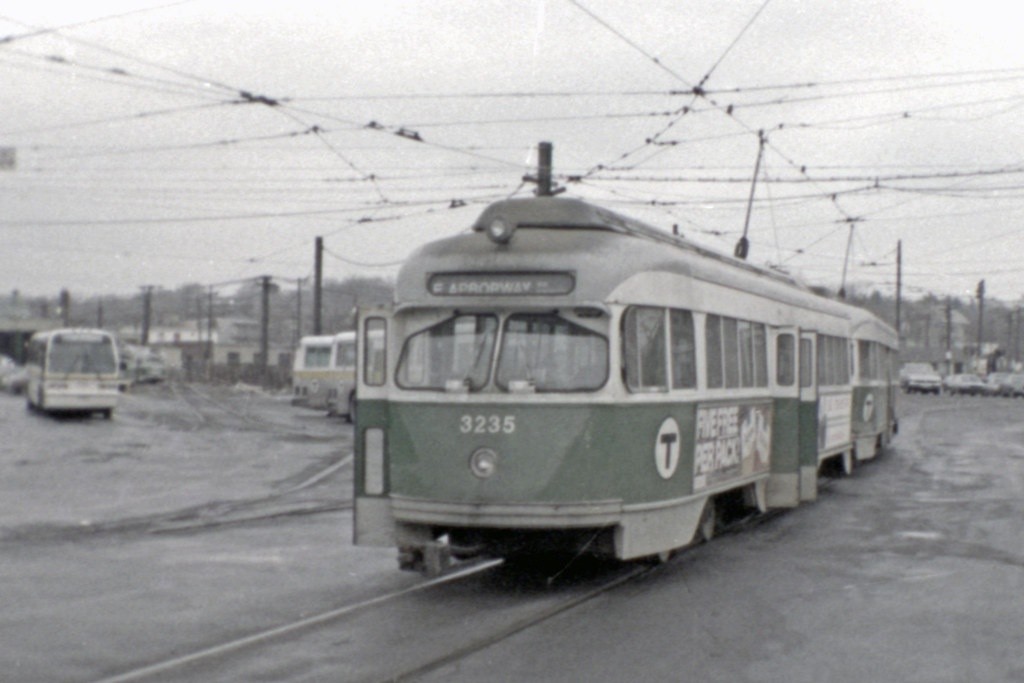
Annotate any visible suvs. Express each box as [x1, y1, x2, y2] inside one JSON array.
[[900, 361, 941, 395]]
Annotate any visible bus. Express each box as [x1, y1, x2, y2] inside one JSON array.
[[290, 325, 500, 426], [23, 327, 120, 419], [341, 192, 904, 575]]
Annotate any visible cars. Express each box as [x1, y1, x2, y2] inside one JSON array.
[[940, 373, 1024, 397]]
